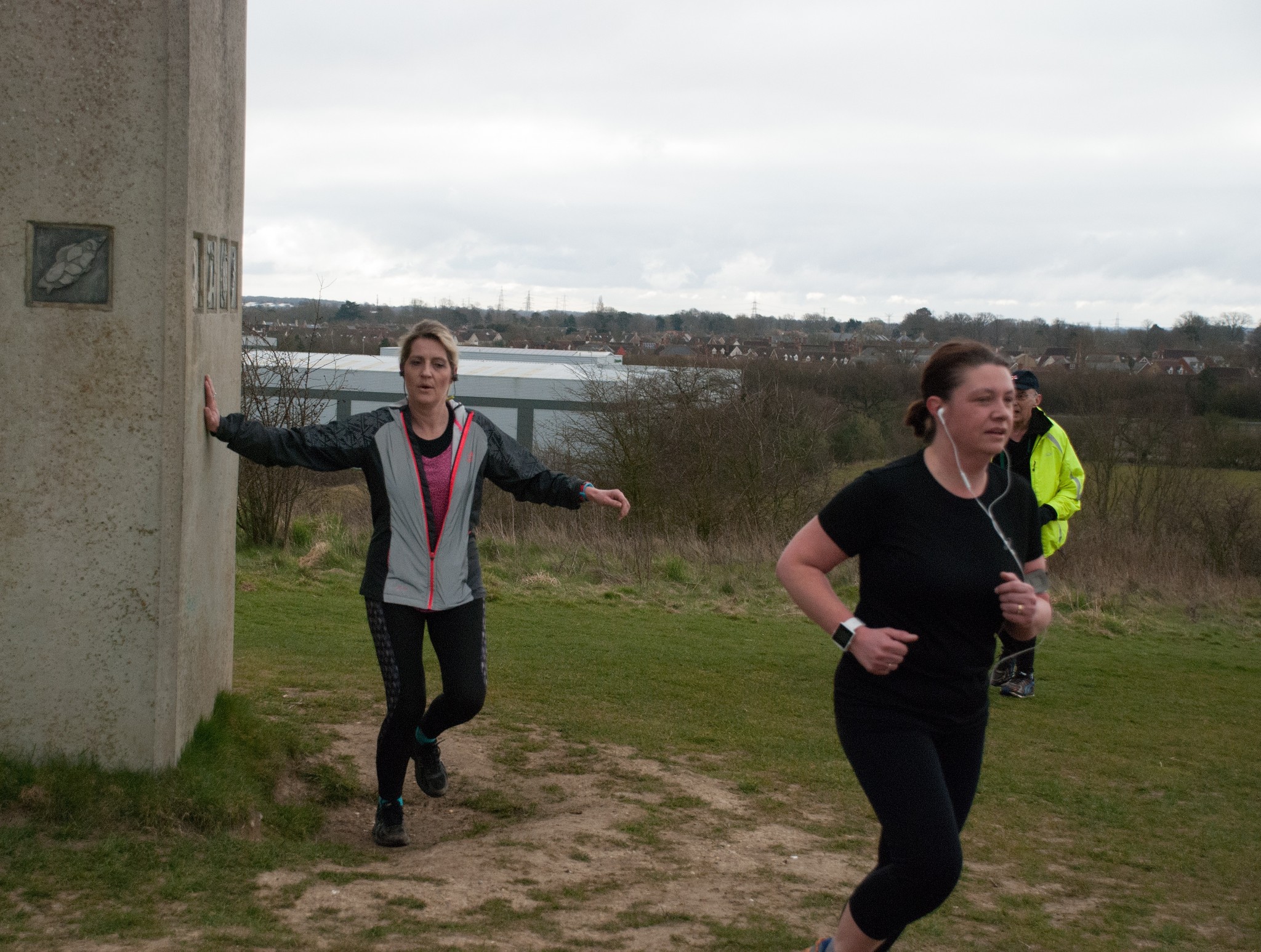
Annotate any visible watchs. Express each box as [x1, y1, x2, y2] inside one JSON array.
[[830, 616, 867, 652]]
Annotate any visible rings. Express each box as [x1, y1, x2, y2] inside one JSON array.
[[1017, 604, 1023, 615], [886, 663, 892, 671]]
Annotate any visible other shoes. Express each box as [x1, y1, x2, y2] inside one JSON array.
[[804, 936, 832, 952]]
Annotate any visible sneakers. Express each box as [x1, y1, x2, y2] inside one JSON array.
[[1000, 670, 1035, 698], [989, 646, 1016, 686], [372, 795, 409, 847], [410, 727, 448, 798]]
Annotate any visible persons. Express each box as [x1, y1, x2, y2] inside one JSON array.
[[990, 370, 1086, 699], [776, 341, 1055, 950], [203, 319, 632, 846]]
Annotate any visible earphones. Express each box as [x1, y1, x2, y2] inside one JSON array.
[[937, 407, 944, 426], [399, 370, 405, 376], [451, 374, 457, 382]]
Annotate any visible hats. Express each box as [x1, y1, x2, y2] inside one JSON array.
[[1011, 370, 1039, 390]]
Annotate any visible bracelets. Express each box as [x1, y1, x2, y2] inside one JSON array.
[[579, 481, 593, 502]]
